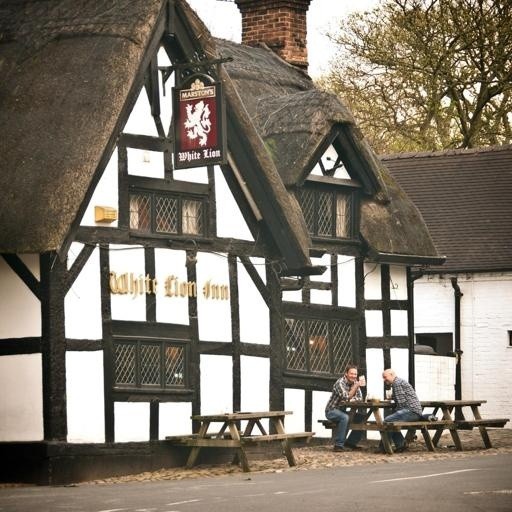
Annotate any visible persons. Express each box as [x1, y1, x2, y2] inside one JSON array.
[[325, 364, 368, 452], [372, 368, 424, 454]]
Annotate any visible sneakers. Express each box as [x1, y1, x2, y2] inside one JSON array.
[[332, 440, 361, 452], [375, 440, 408, 453]]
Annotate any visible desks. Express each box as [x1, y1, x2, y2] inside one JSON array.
[[186, 411, 296, 473], [339, 399, 492, 456]]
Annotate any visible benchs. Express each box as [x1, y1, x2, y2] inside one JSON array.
[[317, 417, 438, 443], [382, 418, 510, 455], [240, 431, 316, 472], [165, 431, 243, 470]]
